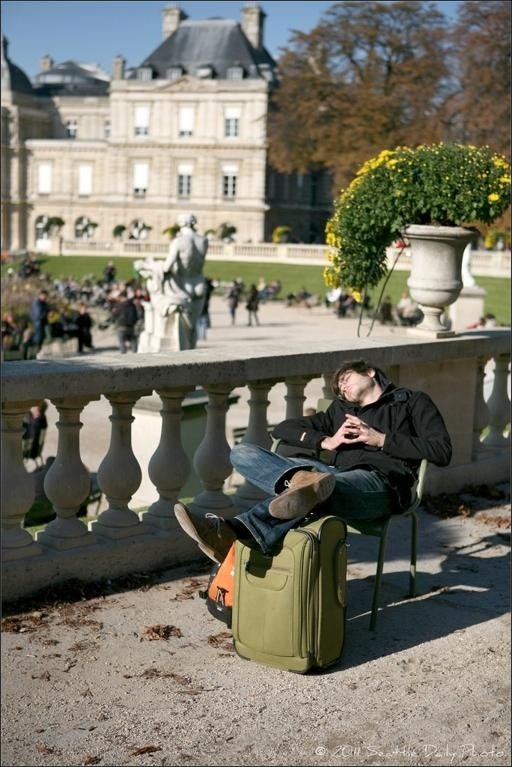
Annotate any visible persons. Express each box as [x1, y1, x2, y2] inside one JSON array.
[[301, 293, 320, 308], [245, 283, 261, 325], [287, 285, 311, 306], [165, 210, 209, 301], [322, 285, 424, 327], [195, 277, 215, 340], [1, 251, 150, 359], [256, 274, 267, 306], [235, 275, 243, 292], [225, 280, 238, 325], [172, 359, 452, 568], [262, 278, 281, 304]]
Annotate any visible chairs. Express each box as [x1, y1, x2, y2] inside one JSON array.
[[345, 459, 428, 631]]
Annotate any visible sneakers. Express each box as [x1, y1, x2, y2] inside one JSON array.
[[268, 470, 335, 520], [173, 502, 236, 565]]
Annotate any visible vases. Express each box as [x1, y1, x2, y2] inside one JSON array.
[[401, 222, 475, 339]]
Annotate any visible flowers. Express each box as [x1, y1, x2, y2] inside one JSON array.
[[324, 142, 512, 303]]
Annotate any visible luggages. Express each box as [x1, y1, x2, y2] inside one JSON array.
[[207, 512, 345, 678]]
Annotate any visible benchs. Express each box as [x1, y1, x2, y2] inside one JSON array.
[[233, 425, 278, 445], [21, 471, 103, 540]]
